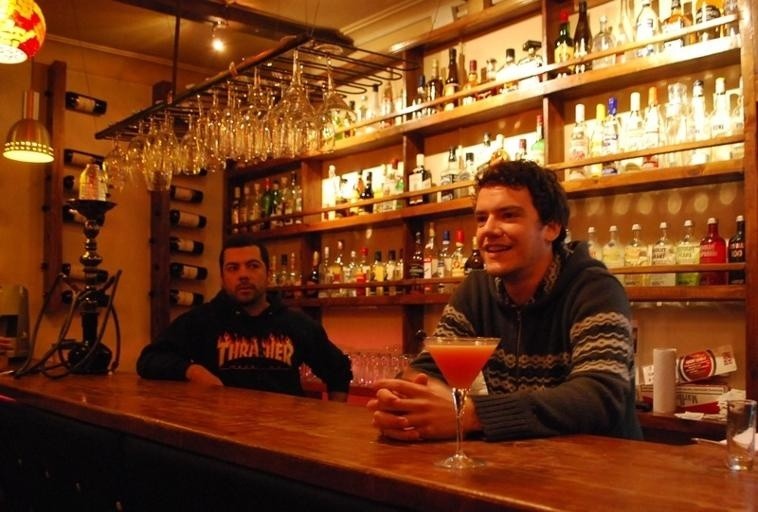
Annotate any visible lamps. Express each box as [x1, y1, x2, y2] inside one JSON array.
[[3, 91, 56, 164]]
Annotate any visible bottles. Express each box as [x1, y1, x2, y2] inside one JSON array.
[[42, 91, 112, 307], [357, 75, 401, 132], [357, 227, 485, 294], [409, 38, 544, 116], [410, 112, 545, 204], [302, 347, 409, 385], [586, 198, 745, 290], [552, 5, 743, 76], [230, 167, 374, 299], [565, 74, 743, 176], [375, 157, 403, 212], [150, 186, 208, 308]]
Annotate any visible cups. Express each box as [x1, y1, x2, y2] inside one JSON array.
[[725, 400, 757, 471]]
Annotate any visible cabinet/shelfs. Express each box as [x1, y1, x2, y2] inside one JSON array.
[[222, 0, 758, 448]]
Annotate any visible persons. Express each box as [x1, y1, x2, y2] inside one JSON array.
[[137, 235, 353, 403], [367, 162, 644, 444]]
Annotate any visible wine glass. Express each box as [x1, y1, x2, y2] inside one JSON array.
[[102, 51, 356, 191], [424, 335, 504, 471]]
